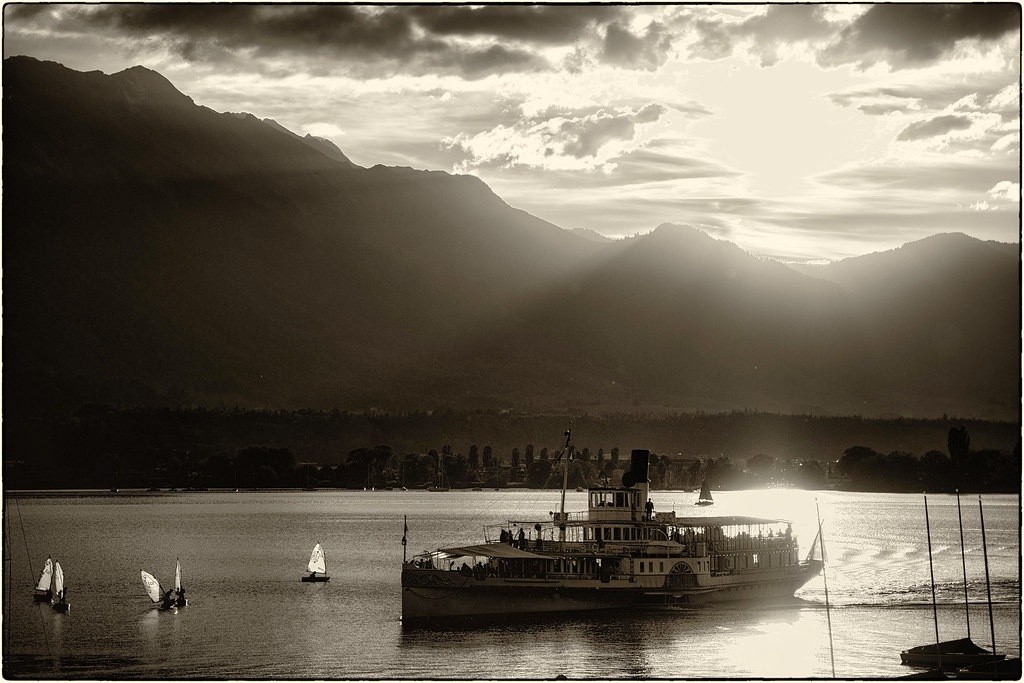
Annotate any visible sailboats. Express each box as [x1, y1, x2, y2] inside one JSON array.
[[53, 560, 71, 613], [695, 476, 714, 506], [428, 462, 450, 492], [34, 555, 53, 603], [139, 569, 177, 612], [472, 476, 482, 491], [494, 469, 499, 491], [301, 543, 330, 583], [402, 468, 407, 491], [173, 557, 187, 607]]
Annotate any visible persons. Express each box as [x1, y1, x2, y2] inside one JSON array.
[[675, 529, 681, 544], [757, 531, 763, 538], [768, 528, 774, 537], [785, 525, 792, 536], [500, 529, 514, 547], [669, 528, 675, 540], [519, 528, 525, 551], [645, 498, 654, 523], [776, 529, 784, 537], [414, 557, 546, 582]]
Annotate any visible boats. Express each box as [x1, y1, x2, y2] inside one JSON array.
[[900, 636, 1005, 667], [577, 487, 584, 492], [401, 428, 825, 629]]
